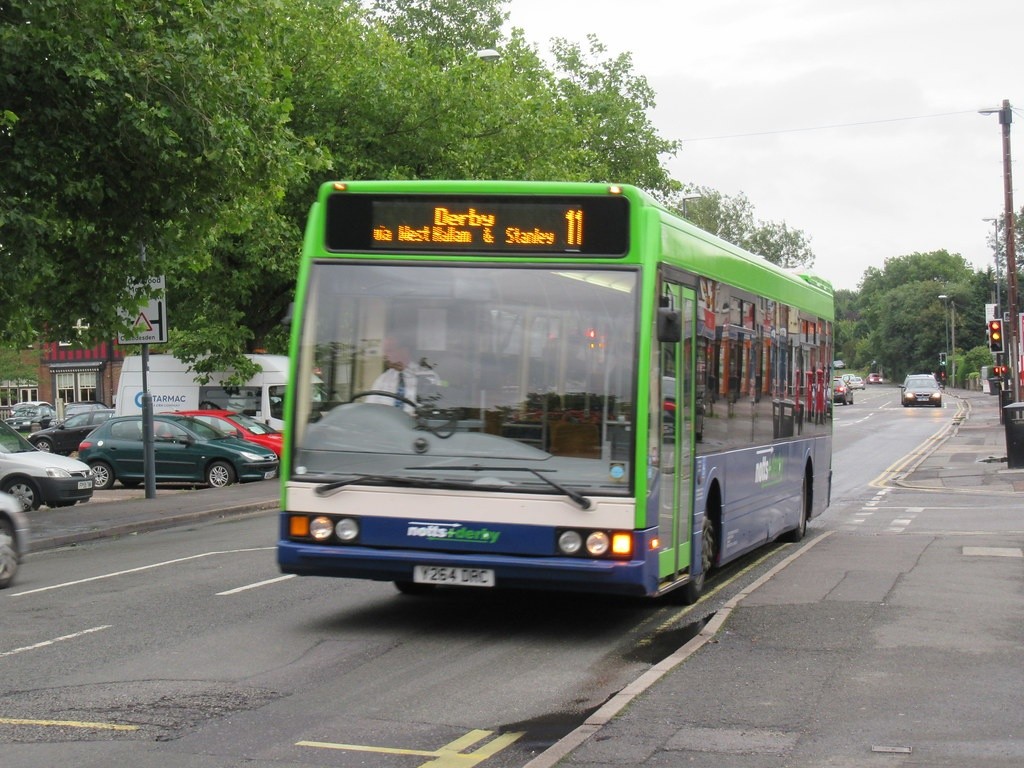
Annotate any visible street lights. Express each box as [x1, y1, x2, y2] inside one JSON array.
[[938, 294, 949, 386], [982, 218, 1005, 365]]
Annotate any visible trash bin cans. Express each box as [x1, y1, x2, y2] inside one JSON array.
[[1003, 401, 1024, 469], [999, 390, 1012, 425], [950, 375, 958, 388], [988, 378, 1000, 394]]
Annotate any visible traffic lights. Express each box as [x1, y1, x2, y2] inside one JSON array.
[[585, 327, 597, 350], [987, 319, 1005, 353], [993, 364, 1008, 377], [939, 353, 947, 366]]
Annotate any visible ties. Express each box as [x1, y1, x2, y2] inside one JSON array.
[[395, 372, 405, 408]]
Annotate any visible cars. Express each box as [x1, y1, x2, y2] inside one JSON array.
[[64, 402, 110, 419], [77, 414, 279, 487], [898, 374, 942, 408], [834, 360, 845, 370], [847, 377, 866, 391], [662, 375, 705, 444], [154, 409, 284, 461], [4, 406, 57, 431], [833, 376, 854, 405], [866, 373, 883, 384], [10, 401, 53, 415], [27, 408, 117, 457], [841, 374, 855, 383], [0, 490, 32, 590]]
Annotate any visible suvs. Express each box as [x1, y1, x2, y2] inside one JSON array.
[[0, 416, 94, 513]]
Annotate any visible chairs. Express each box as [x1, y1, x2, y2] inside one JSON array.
[[549, 410, 600, 459]]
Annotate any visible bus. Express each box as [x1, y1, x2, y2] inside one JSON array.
[[272, 179, 834, 606]]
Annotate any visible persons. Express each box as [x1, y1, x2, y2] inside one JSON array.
[[364, 328, 449, 417]]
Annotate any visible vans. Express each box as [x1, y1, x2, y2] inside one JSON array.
[[114, 352, 347, 436]]
